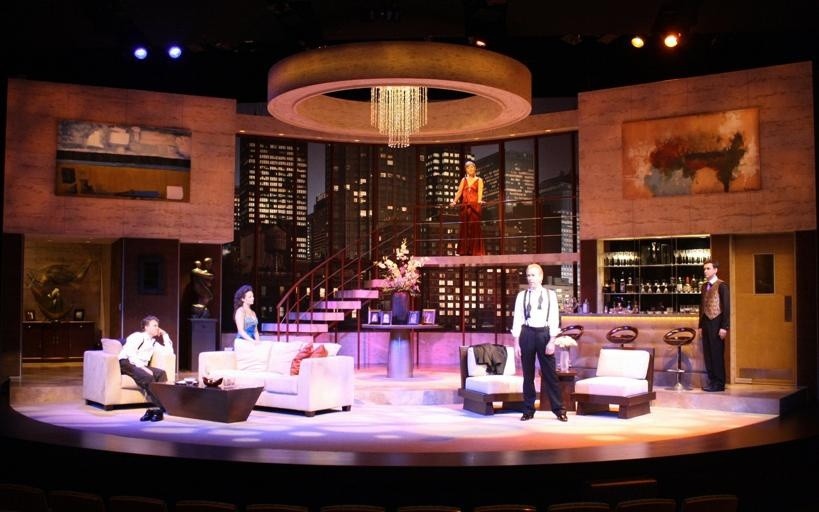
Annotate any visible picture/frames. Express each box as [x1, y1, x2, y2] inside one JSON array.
[[368, 309, 381, 325], [381, 311, 392, 325], [407, 310, 420, 324], [422, 309, 436, 325]]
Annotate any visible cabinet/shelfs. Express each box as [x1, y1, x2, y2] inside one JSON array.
[[580, 234, 712, 315]]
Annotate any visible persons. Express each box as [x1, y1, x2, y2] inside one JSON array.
[[449, 158, 485, 256], [233, 285, 260, 341], [119, 315, 174, 415], [510, 263, 569, 422], [697, 260, 730, 392]]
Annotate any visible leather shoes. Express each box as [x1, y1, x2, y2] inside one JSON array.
[[521, 414, 533, 420], [703, 386, 724, 391], [559, 414, 567, 421], [141, 410, 163, 421]]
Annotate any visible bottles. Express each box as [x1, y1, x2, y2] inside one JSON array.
[[577, 297, 589, 314], [604, 267, 639, 293], [677, 274, 708, 293], [604, 296, 638, 314]]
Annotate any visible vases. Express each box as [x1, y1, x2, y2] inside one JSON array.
[[560, 350, 570, 373], [391, 290, 410, 324]]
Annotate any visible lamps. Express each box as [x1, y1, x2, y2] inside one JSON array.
[[371, 86, 428, 149]]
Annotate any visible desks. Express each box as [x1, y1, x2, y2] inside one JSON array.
[[187, 318, 219, 372], [20, 321, 96, 361], [362, 324, 444, 379]]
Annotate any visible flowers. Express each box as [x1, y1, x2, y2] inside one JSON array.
[[641, 127, 747, 196], [553, 336, 578, 352], [373, 236, 424, 297]]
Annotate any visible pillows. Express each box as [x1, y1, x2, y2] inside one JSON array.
[[233, 338, 341, 376], [101, 337, 123, 354]]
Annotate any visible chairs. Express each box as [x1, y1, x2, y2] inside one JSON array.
[[458, 345, 541, 416], [84, 351, 176, 411], [663, 327, 696, 391], [570, 346, 656, 419], [607, 326, 638, 349]]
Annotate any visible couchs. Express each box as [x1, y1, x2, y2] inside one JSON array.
[[198, 350, 354, 417]]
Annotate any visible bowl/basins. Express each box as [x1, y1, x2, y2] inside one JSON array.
[[184, 378, 197, 385]]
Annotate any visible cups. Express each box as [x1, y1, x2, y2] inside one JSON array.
[[223, 373, 236, 386], [642, 306, 673, 314], [678, 305, 700, 314]]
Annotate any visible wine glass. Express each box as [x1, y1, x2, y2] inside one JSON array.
[[563, 298, 575, 314], [605, 251, 637, 265], [673, 248, 711, 265], [640, 280, 670, 294]]
